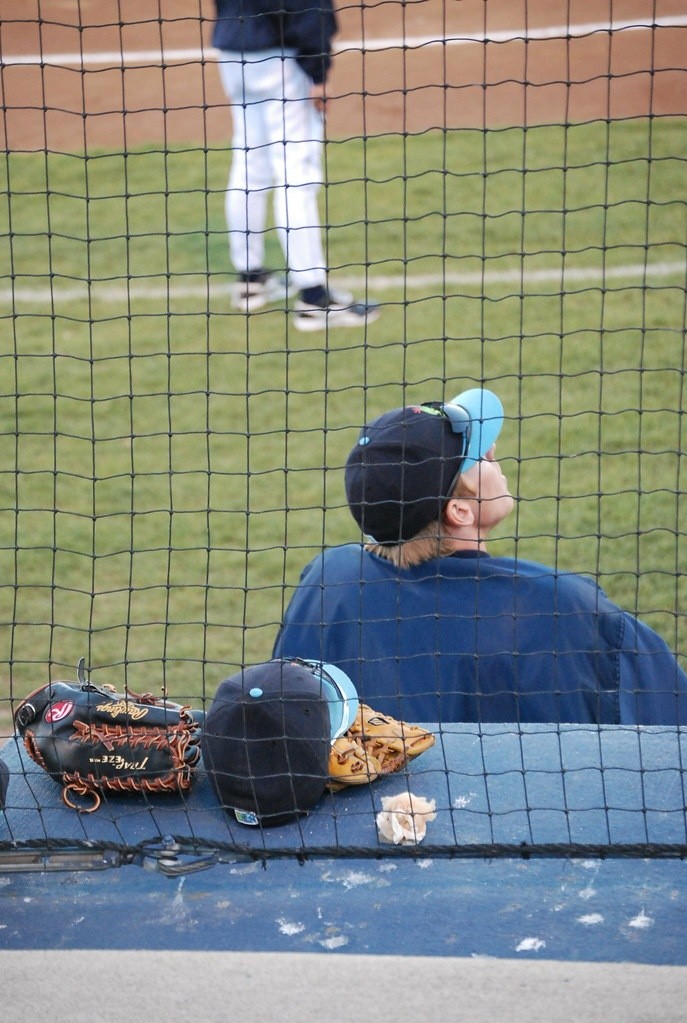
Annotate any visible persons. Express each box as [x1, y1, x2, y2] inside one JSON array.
[[211, 0, 383, 332], [272, 388, 686, 724]]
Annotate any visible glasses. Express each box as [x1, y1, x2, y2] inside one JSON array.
[[420, 401, 470, 522]]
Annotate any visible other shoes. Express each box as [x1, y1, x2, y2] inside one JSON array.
[[292, 289, 381, 332], [231, 272, 301, 312]]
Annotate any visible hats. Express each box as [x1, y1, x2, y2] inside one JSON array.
[[344, 389, 504, 546], [201, 657, 359, 830]]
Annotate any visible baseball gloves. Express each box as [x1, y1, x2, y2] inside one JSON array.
[[327, 704, 435, 796], [13, 656, 208, 813]]
